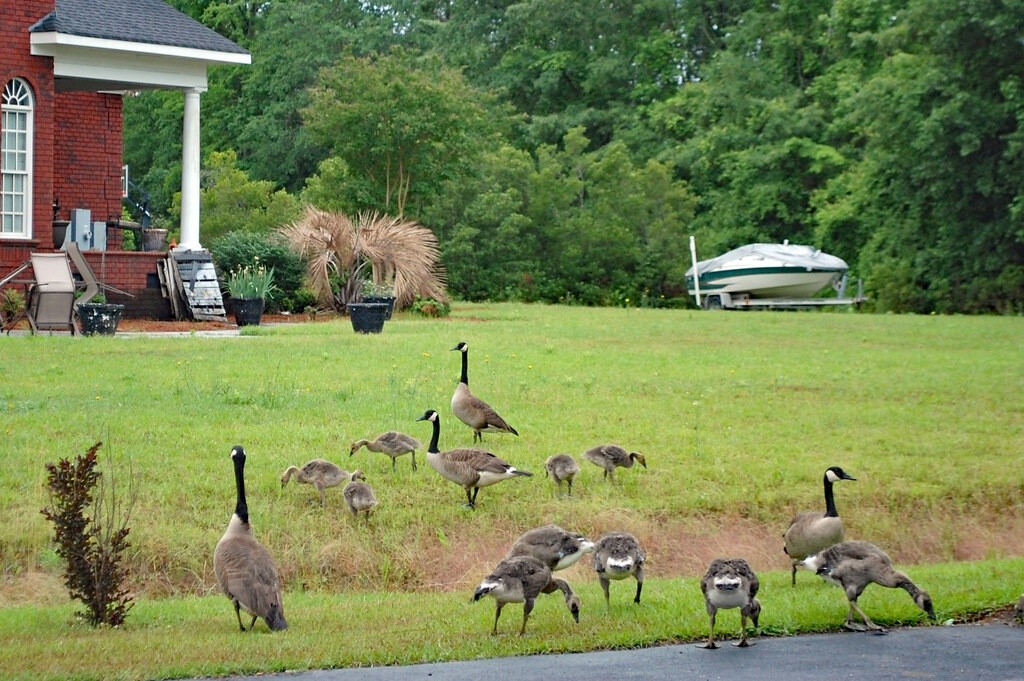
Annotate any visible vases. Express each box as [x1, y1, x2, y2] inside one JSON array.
[[76, 302, 123, 336], [348, 303, 388, 333], [144, 228, 167, 252], [228, 297, 262, 326], [363, 296, 395, 321], [53, 220, 72, 248]]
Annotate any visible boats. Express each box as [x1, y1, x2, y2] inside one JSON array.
[[684, 236, 868, 313]]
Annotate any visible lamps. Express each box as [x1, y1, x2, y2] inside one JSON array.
[[124, 88, 141, 97]]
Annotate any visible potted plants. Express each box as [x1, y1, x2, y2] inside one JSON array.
[[0, 288, 24, 323]]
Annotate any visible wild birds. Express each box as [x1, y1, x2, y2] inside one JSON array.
[[281, 459, 350, 504], [450, 342, 519, 445], [415, 410, 534, 509], [471, 525, 645, 638], [213, 445, 288, 632], [584, 444, 646, 484], [694, 558, 761, 647], [802, 541, 937, 632], [343, 470, 377, 529], [545, 452, 580, 496], [350, 432, 423, 471], [784, 467, 858, 590]]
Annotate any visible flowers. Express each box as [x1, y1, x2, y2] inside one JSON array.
[[360, 278, 394, 297], [218, 256, 284, 302]]
